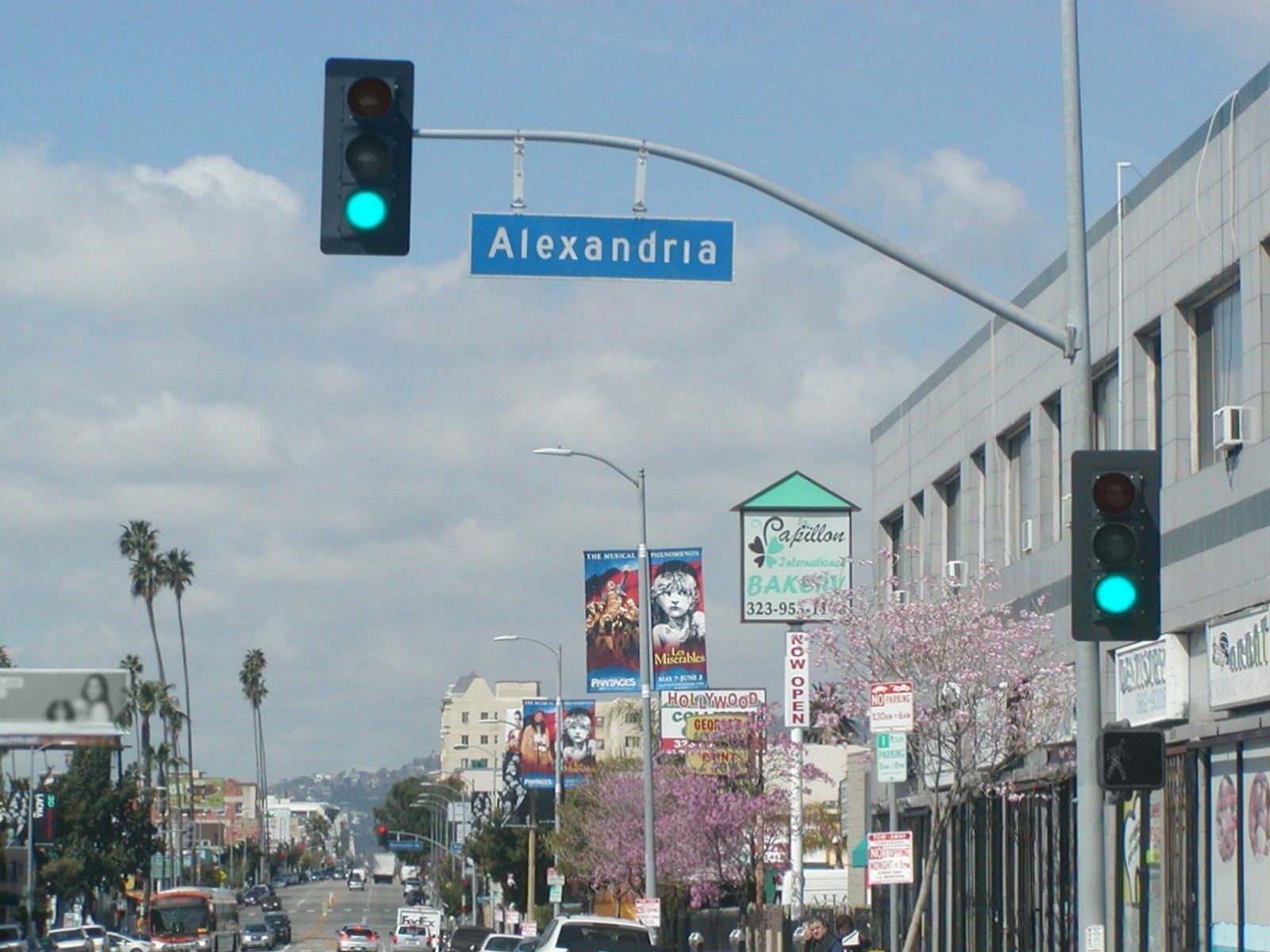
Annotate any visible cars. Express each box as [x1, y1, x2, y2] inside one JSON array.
[[0, 923, 150, 952], [240, 885, 291, 952], [273, 867, 368, 892], [439, 914, 659, 952], [389, 866, 445, 951]]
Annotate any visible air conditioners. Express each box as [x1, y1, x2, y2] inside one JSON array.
[[946, 561, 961, 588], [1213, 406, 1243, 450], [893, 590, 906, 606]]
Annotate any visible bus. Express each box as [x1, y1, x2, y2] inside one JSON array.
[[146, 886, 241, 952]]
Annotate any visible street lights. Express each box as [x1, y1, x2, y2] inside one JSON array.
[[491, 634, 562, 920], [26, 741, 79, 924], [409, 720, 502, 926], [534, 446, 660, 948]]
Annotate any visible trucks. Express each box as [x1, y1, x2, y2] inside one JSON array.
[[370, 854, 398, 883]]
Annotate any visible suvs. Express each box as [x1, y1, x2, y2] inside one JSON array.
[[335, 923, 381, 950]]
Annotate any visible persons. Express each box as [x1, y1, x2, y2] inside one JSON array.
[[734, 926, 745, 952], [805, 916, 843, 952], [773, 873, 782, 905]]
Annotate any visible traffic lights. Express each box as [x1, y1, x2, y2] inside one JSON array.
[[1098, 729, 1169, 789], [376, 825, 388, 849], [280, 851, 285, 861], [320, 57, 416, 255], [1069, 449, 1161, 641]]
